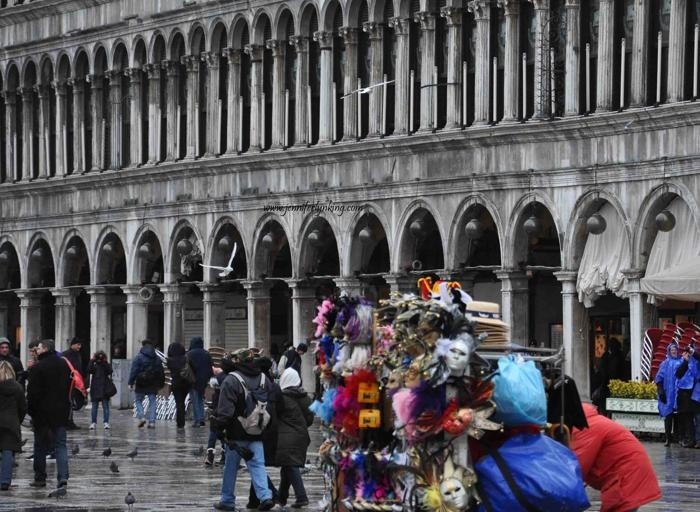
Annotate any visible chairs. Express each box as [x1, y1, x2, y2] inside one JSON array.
[[641, 323, 699, 394], [133, 339, 227, 420]]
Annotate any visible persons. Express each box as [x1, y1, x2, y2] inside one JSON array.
[[0, 329, 328, 512], [654, 342, 700, 449], [567, 400, 664, 512]]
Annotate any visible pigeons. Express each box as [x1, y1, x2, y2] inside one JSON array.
[[101, 447, 112, 459], [124, 491, 136, 510], [48, 481, 69, 501], [127, 446, 139, 461], [109, 461, 119, 474], [71, 443, 81, 456], [191, 445, 204, 458]]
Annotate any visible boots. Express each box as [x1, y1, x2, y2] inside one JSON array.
[[664, 439, 669, 447], [66, 419, 81, 431], [204, 447, 226, 466]]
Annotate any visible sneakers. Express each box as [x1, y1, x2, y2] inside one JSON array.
[[30, 480, 46, 487], [45, 453, 57, 463], [25, 454, 35, 461], [137, 418, 146, 427], [246, 488, 288, 511], [104, 422, 110, 429], [677, 439, 699, 448], [147, 421, 155, 428], [88, 422, 96, 429], [177, 427, 184, 431], [56, 479, 67, 488], [192, 423, 200, 428], [213, 501, 236, 512], [0, 482, 10, 491], [291, 499, 309, 507], [200, 421, 205, 426]]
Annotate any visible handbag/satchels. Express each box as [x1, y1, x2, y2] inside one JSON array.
[[179, 353, 197, 385], [102, 373, 117, 398], [135, 353, 158, 395]]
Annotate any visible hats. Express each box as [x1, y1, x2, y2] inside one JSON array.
[[0, 337, 10, 344], [298, 343, 308, 352], [71, 337, 83, 345], [142, 339, 152, 346], [237, 349, 254, 362], [99, 350, 103, 355]]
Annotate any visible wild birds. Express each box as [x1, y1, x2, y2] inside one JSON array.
[[338, 79, 396, 101], [196, 241, 239, 282]]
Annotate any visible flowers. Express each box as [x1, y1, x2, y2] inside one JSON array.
[[606, 374, 663, 401]]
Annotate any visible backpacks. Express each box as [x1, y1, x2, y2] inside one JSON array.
[[60, 355, 88, 411], [228, 371, 272, 436]]
